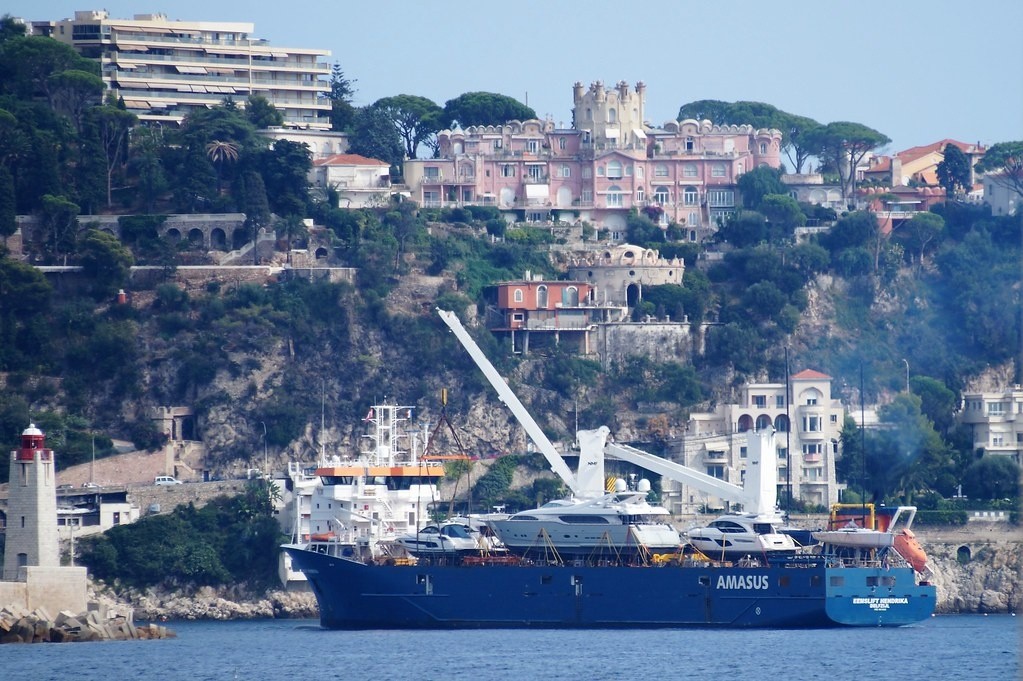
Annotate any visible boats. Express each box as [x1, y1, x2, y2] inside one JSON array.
[[393, 516, 506, 558], [277, 311, 937, 629], [480, 491, 681, 563], [812, 526, 895, 549], [686, 516, 802, 562]]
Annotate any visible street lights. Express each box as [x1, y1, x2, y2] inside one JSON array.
[[902, 358, 909, 393]]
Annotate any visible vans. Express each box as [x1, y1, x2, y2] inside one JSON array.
[[154, 477, 182, 486], [81, 483, 101, 488], [56, 485, 73, 490]]
